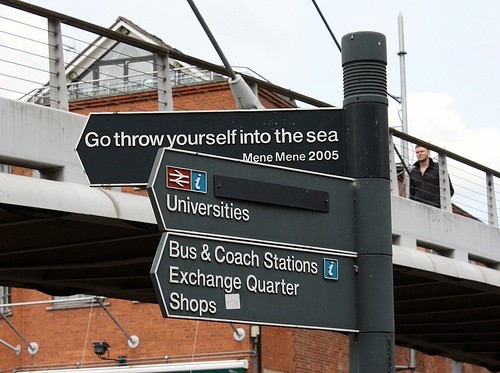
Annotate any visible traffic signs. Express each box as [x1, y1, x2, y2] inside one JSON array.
[[78, 104, 360, 334]]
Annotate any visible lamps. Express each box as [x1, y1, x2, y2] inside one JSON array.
[[91, 341, 111, 355]]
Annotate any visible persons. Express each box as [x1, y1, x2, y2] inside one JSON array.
[[409, 141, 454, 209]]
